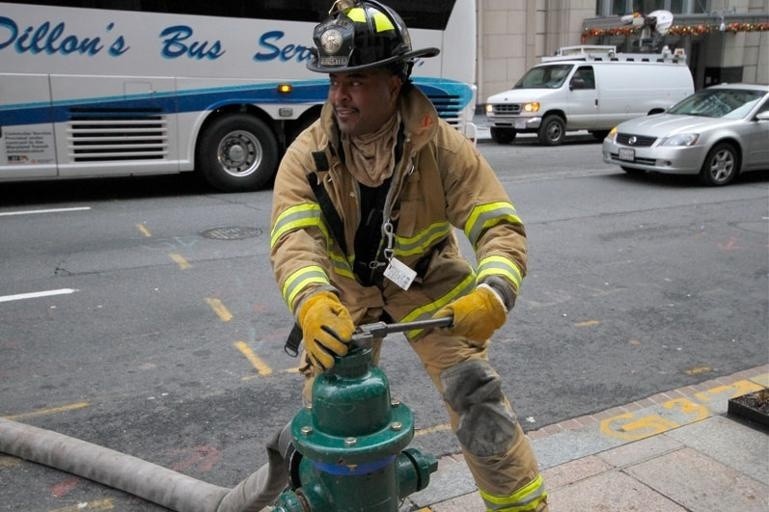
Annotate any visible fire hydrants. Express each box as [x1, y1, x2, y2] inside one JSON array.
[[268, 325, 440, 511]]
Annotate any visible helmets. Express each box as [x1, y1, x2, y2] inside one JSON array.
[[306, 0, 440, 74]]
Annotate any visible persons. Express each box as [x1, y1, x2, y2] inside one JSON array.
[[271, 1, 547, 512]]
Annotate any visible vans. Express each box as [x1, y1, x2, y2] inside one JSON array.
[[485, 44, 698, 147]]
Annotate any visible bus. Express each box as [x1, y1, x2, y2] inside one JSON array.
[[0, 0, 480, 191]]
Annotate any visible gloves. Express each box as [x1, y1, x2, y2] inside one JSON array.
[[431, 284, 509, 348], [298, 291, 355, 375]]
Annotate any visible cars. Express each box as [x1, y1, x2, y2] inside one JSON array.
[[601, 83, 769, 186]]
